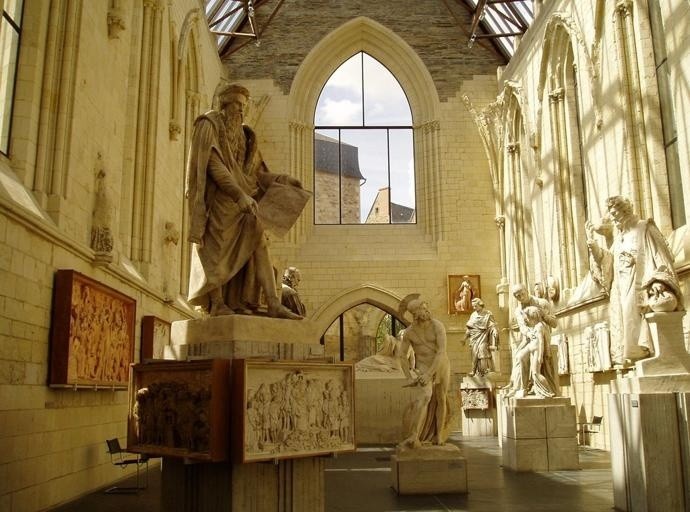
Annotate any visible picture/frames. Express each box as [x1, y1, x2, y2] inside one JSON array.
[[447, 274, 481, 314], [49, 269, 171, 390], [230, 358, 357, 464], [126, 357, 228, 463]]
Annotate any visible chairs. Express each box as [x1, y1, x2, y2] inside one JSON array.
[[105, 438, 148, 494]]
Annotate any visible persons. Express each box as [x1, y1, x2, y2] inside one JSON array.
[[186, 82, 305, 320], [278, 267, 307, 318], [503, 286, 562, 398], [398, 300, 450, 446], [460, 298, 497, 377], [459, 275, 472, 310], [514, 307, 556, 397], [585, 195, 682, 368]]
